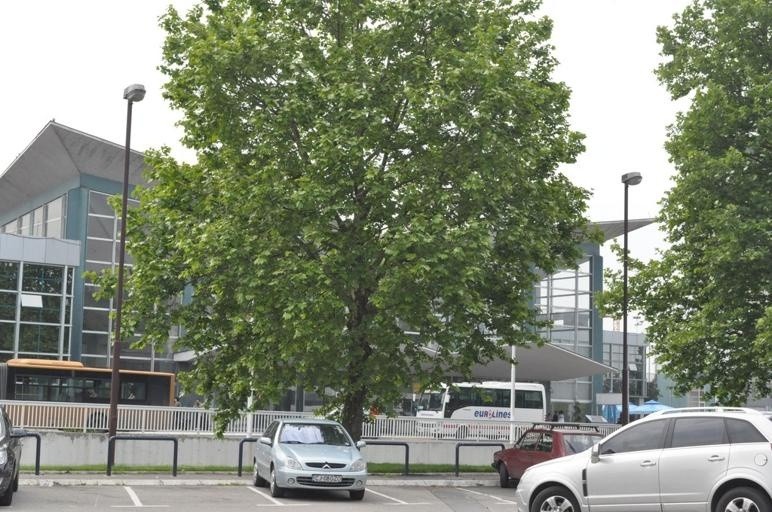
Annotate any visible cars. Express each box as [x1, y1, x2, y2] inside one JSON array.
[[0, 406, 22, 505], [253, 418, 368, 500], [491, 407, 772, 512]]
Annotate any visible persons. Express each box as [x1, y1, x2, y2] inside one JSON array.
[[552, 411, 558, 422], [88, 388, 97, 399], [546, 412, 553, 421], [126, 389, 134, 400], [557, 410, 566, 422], [192, 398, 203, 408], [173, 396, 181, 407]]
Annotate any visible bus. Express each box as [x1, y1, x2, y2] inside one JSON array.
[[0, 359, 175, 434], [416, 381, 546, 439]]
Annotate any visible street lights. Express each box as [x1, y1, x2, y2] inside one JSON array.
[[621, 172, 643, 427], [106, 84, 146, 475]]
